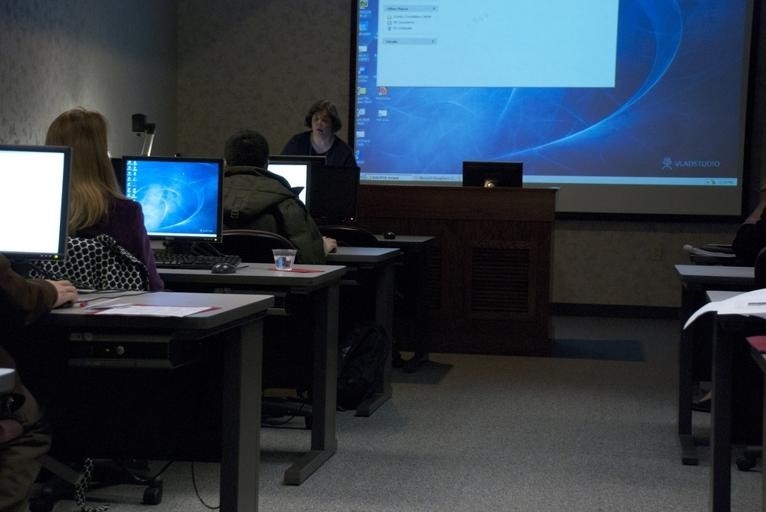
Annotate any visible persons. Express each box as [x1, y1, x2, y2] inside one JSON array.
[[222, 129, 338, 264], [281, 100, 357, 220], [0, 253, 79, 512], [731, 178, 766, 262], [44, 105, 165, 291]]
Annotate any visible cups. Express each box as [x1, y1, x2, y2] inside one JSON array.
[[272, 248, 297, 271]]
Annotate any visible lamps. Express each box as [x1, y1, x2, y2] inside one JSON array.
[[132, 113, 157, 157]]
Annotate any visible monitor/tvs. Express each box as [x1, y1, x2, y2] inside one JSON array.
[[0, 144, 74, 260], [463, 161, 523, 187], [311, 165, 361, 227], [268, 161, 311, 212], [121, 155, 224, 244], [269, 155, 328, 165]]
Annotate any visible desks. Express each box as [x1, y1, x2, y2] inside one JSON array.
[[326, 246, 400, 417], [706, 290, 766, 512], [673, 263, 756, 466], [156, 262, 347, 486], [49, 289, 275, 512], [372, 234, 435, 373]]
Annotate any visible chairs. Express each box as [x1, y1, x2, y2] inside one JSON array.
[[210, 229, 311, 429], [319, 227, 379, 247], [27, 230, 162, 505]]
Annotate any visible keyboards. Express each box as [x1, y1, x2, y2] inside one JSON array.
[[153, 252, 240, 270]]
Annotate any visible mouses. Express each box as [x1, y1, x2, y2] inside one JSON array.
[[384, 231, 396, 239], [211, 263, 236, 274], [53, 300, 73, 309]]
